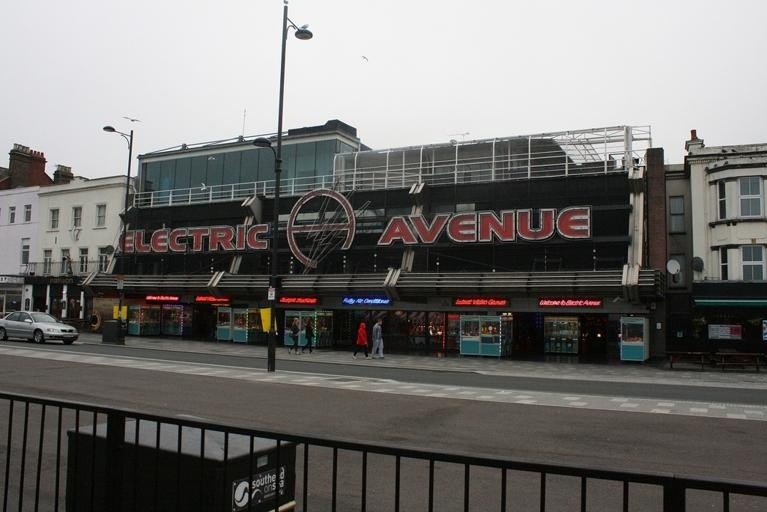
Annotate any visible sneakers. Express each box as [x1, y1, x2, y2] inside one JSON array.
[[352, 354, 385, 361], [288, 347, 313, 356]]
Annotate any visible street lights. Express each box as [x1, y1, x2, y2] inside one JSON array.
[[252, 2, 314, 371], [104, 126, 134, 345]]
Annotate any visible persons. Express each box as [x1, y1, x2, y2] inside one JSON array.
[[352, 323, 372, 359], [301, 320, 314, 353], [372, 318, 384, 359], [288, 318, 300, 354]]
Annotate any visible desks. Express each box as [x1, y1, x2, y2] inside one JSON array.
[[664, 350, 711, 369], [714, 352, 764, 372]]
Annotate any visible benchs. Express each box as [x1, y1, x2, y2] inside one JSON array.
[[716, 362, 767, 373], [670, 360, 711, 368]]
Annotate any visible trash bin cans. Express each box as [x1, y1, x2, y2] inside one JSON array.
[[65, 413, 301, 512], [102, 320, 126, 346]]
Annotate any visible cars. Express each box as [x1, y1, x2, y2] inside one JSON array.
[[0, 311, 79, 345]]
